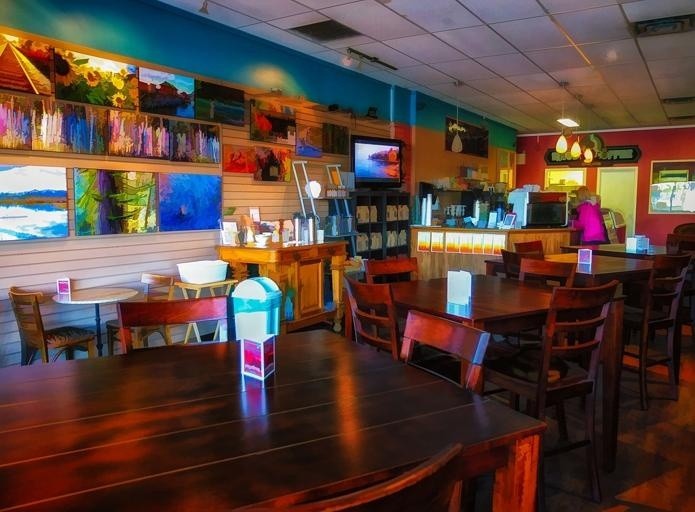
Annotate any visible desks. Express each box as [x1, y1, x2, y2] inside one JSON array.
[[540, 251, 655, 287], [52, 289, 138, 359], [173, 279, 236, 343], [392, 274, 635, 391]]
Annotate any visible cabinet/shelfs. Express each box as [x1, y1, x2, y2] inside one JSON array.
[[216, 241, 346, 333]]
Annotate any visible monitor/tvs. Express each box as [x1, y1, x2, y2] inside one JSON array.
[[350, 135, 403, 191]]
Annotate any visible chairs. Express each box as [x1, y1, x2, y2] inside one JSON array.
[[363, 257, 418, 285], [518, 254, 578, 291], [482, 280, 621, 506], [9, 287, 97, 367], [107, 272, 173, 353], [616, 254, 688, 414], [500, 249, 544, 282], [291, 440, 467, 512], [514, 241, 546, 257], [340, 274, 397, 362], [399, 308, 492, 391]]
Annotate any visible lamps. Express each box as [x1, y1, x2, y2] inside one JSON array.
[[450, 90, 465, 154], [197, 1, 212, 17], [584, 138, 593, 163], [556, 116, 582, 130], [555, 109, 569, 153], [570, 125, 581, 161]]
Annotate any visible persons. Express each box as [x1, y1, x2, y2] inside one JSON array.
[[569, 186, 610, 245]]
